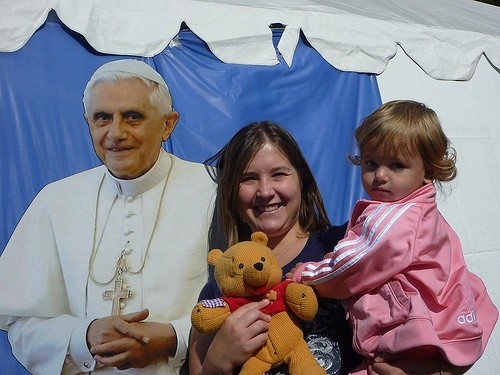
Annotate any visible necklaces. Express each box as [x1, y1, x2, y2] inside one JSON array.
[[276, 228, 304, 259], [88, 153, 173, 317]]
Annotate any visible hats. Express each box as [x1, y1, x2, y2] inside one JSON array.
[[91, 58, 170, 90]]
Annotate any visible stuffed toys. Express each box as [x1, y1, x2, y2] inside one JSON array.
[[191, 230, 327, 375]]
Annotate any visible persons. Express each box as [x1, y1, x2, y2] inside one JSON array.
[[285, 100, 499, 375], [178, 120, 476, 374], [0, 59, 239, 375]]
